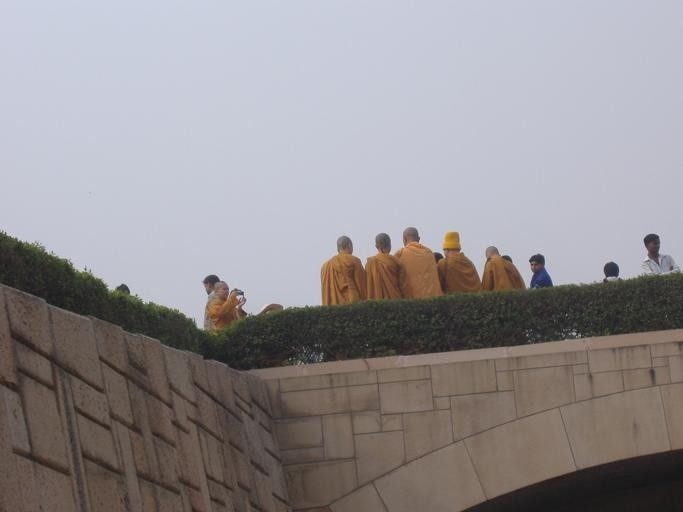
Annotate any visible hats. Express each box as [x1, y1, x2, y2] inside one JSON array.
[[443, 231, 460, 250]]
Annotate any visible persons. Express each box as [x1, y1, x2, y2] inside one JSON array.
[[603, 262, 623, 283], [529, 254, 553, 289], [438, 232, 483, 294], [320, 235, 365, 306], [433, 252, 443, 264], [640, 234, 680, 276], [502, 255, 513, 263], [482, 246, 525, 291], [394, 227, 444, 298], [207, 281, 247, 330], [116, 284, 130, 295], [204, 275, 220, 331], [364, 233, 408, 301]]
